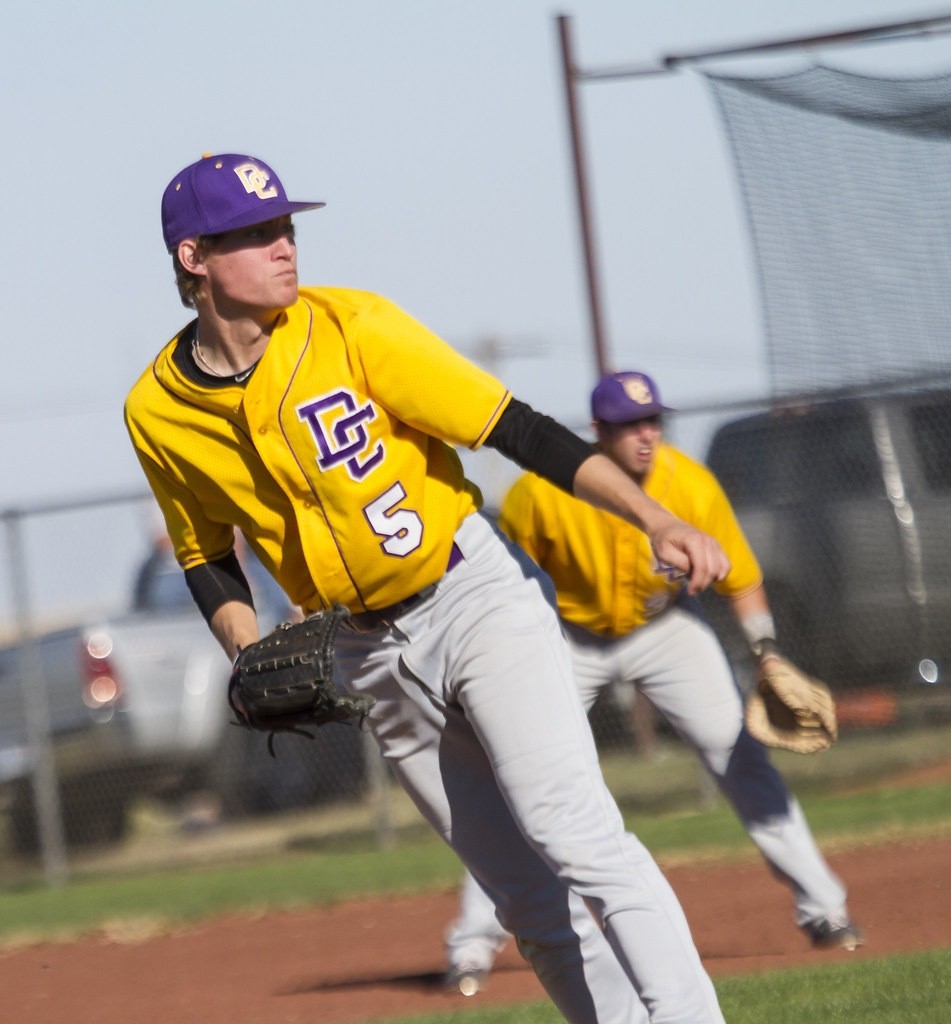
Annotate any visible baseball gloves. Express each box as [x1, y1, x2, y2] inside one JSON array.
[[225, 604, 389, 733], [742, 655, 839, 755]]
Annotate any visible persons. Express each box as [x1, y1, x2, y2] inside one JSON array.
[[123, 153, 725, 1024], [439, 371, 856, 990]]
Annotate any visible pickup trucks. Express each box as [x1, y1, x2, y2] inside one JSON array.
[[2, 530, 378, 855]]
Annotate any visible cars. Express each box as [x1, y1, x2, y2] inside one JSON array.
[[701, 383, 949, 692]]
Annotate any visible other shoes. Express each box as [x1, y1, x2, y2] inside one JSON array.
[[811, 923, 863, 947], [441, 959, 487, 994]]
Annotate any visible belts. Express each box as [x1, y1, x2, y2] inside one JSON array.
[[355, 540, 463, 627]]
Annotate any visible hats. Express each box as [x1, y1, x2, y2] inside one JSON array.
[[162, 153, 326, 251], [591, 372, 679, 424]]
